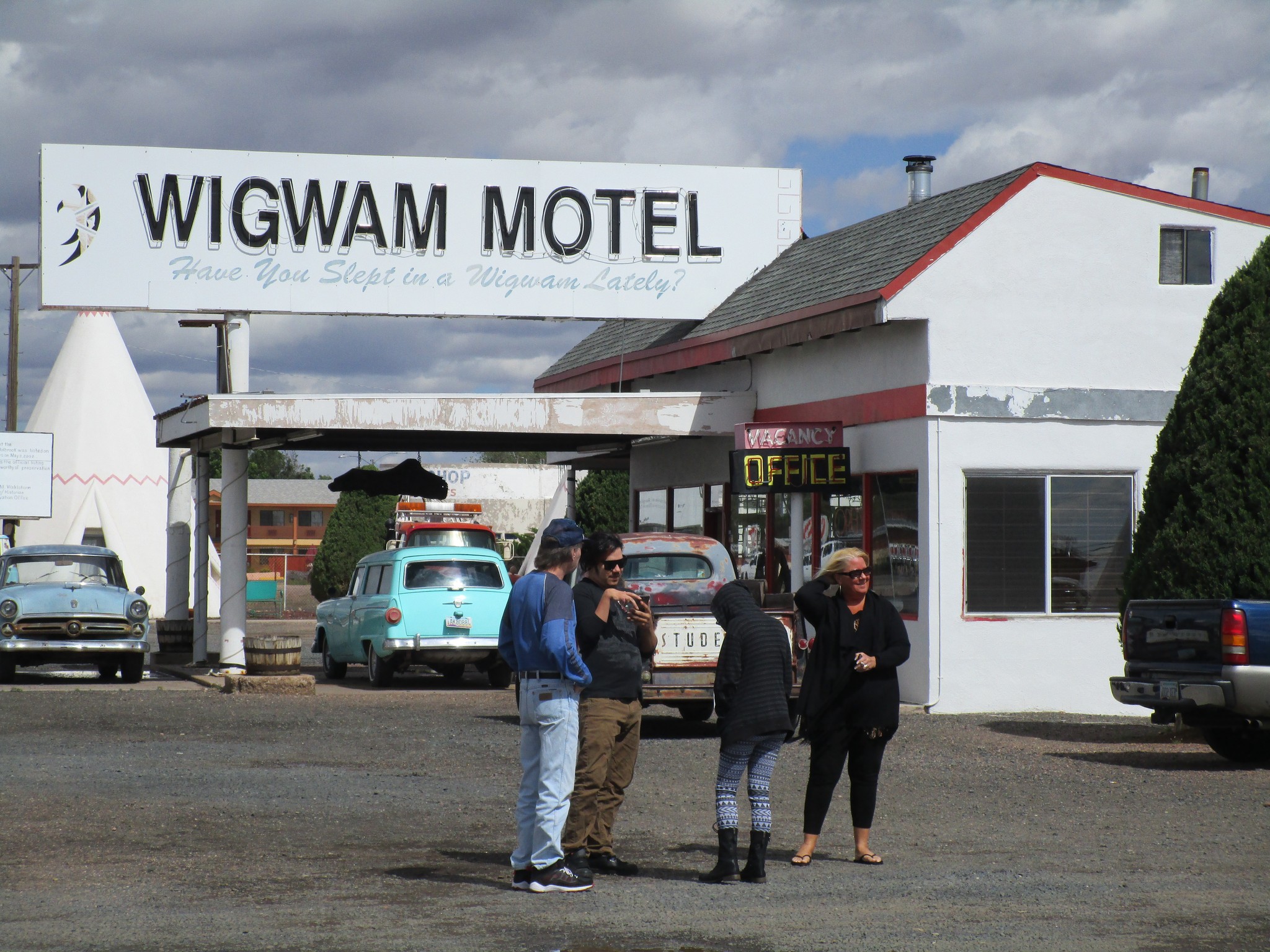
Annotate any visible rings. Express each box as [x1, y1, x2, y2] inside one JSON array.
[[864, 664, 868, 668]]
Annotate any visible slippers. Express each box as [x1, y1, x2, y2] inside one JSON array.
[[853, 852, 884, 866], [791, 854, 812, 865]]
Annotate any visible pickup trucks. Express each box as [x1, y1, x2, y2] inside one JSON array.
[[578, 532, 809, 742], [1109, 600, 1270, 757]]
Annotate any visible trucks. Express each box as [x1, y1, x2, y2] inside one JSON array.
[[801, 522, 924, 586]]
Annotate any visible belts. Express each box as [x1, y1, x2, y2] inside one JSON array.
[[518, 671, 571, 681]]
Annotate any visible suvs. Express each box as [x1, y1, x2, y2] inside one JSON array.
[[310, 544, 515, 690]]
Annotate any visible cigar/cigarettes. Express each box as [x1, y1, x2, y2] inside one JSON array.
[[860, 662, 865, 666]]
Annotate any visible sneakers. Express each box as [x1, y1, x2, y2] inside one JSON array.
[[566, 847, 593, 879], [529, 857, 593, 893], [510, 865, 532, 891], [586, 853, 638, 877]]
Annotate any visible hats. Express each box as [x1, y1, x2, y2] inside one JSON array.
[[538, 517, 593, 552]]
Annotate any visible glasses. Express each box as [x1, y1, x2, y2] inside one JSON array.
[[596, 551, 631, 571], [834, 566, 872, 579]]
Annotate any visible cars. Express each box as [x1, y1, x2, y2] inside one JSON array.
[[738, 551, 789, 581], [0, 545, 151, 684]]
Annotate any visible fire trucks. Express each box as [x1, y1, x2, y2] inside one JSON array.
[[385, 500, 515, 562]]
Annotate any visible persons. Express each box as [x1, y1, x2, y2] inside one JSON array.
[[559, 528, 659, 880], [497, 517, 595, 893], [789, 546, 913, 866], [697, 579, 795, 884]]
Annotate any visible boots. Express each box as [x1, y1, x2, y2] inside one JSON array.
[[698, 827, 741, 884], [740, 830, 771, 883]]
